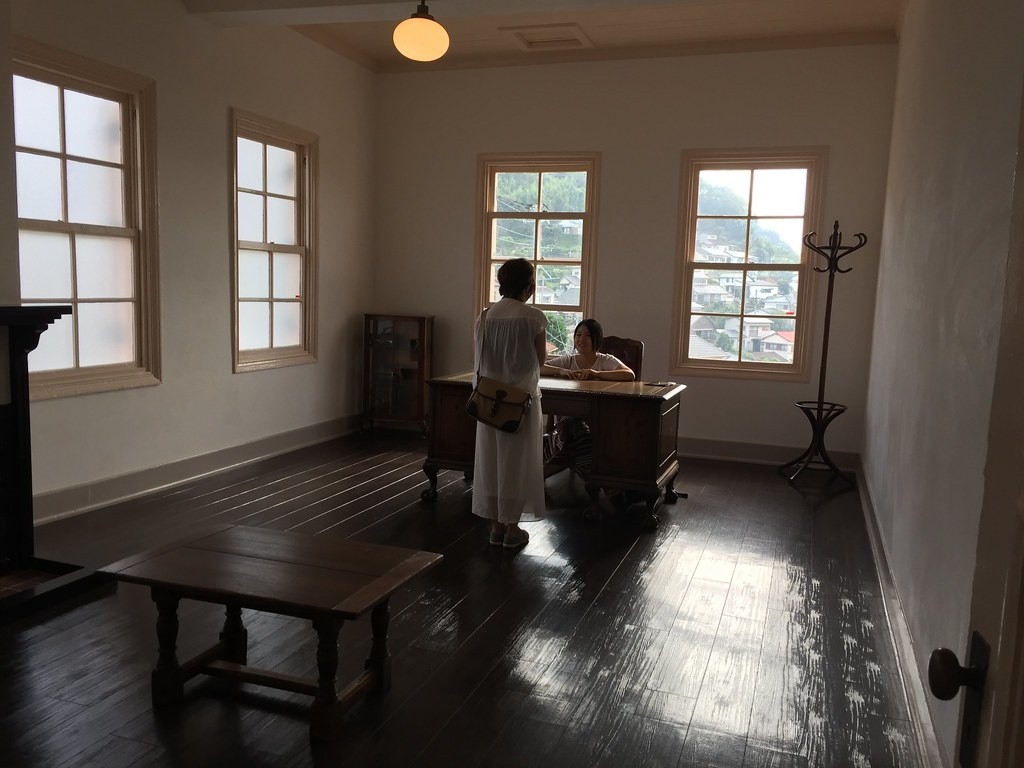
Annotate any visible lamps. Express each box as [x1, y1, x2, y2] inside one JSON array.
[[392, 0, 450, 62]]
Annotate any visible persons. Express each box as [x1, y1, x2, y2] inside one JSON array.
[[540, 318, 635, 504], [470, 258, 547, 548]]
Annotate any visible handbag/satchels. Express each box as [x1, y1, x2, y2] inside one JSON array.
[[464, 376, 532, 434]]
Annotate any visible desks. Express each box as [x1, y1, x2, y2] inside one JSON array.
[[95, 523, 445, 741], [421, 371, 688, 530]]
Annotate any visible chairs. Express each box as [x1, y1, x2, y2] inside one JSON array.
[[546, 336, 644, 433]]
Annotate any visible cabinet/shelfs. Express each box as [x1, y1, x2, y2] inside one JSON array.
[[361, 312, 434, 437]]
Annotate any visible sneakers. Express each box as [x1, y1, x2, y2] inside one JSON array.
[[503, 529, 528, 548], [489, 524, 508, 545]]
